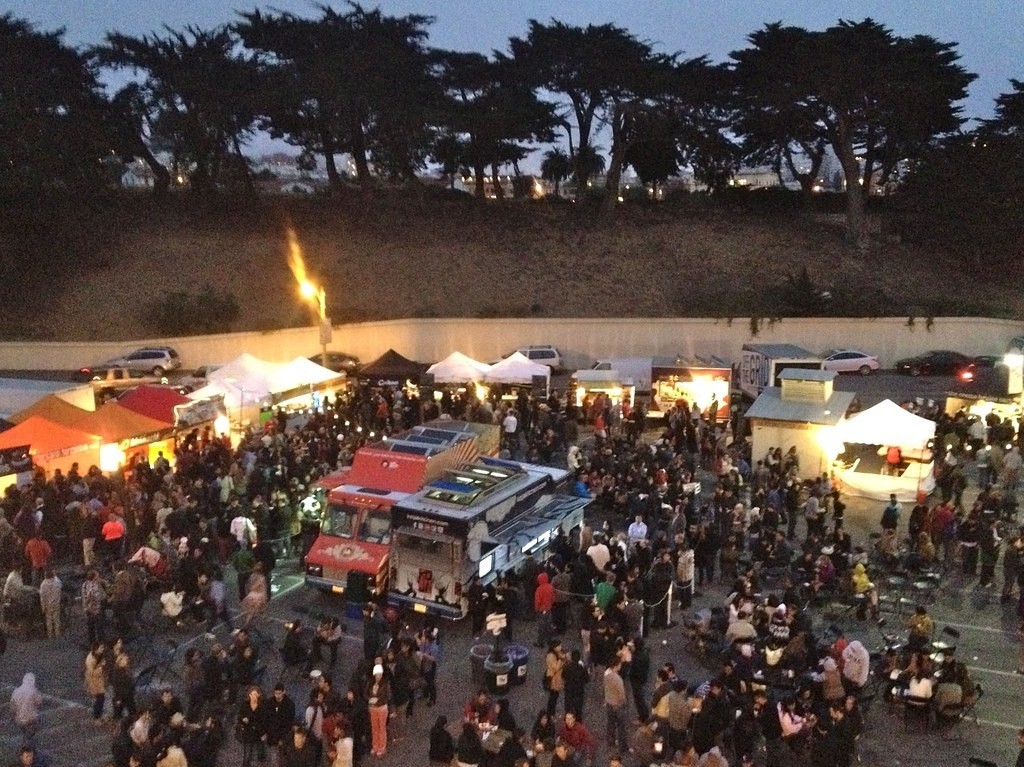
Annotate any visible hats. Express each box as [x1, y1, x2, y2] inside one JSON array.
[[371, 664, 383, 676]]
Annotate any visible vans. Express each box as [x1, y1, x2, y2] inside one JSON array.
[[569, 357, 651, 397]]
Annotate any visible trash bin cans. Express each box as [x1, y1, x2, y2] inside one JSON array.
[[485, 654, 514, 696], [469, 644, 496, 685], [501, 645, 530, 685]]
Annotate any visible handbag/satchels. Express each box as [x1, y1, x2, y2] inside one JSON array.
[[542, 669, 552, 689], [243, 518, 249, 540]]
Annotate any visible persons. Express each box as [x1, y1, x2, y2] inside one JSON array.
[[0, 382, 1024, 767]]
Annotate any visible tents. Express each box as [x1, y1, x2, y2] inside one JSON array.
[[830, 397, 939, 502], [0, 353, 347, 494], [356, 347, 422, 385], [424, 349, 492, 384], [484, 350, 552, 403]]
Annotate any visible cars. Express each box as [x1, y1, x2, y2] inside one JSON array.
[[823, 346, 881, 378], [107, 344, 179, 376], [894, 348, 974, 378], [302, 350, 357, 375], [489, 345, 559, 370]]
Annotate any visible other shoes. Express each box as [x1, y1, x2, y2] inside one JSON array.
[[204, 633, 215, 638], [231, 629, 242, 636]]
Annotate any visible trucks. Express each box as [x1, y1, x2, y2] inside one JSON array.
[[302, 418, 504, 602], [378, 457, 583, 628]]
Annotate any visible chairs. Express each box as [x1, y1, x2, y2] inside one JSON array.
[[280, 648, 310, 683], [878, 627, 984, 732], [113, 622, 179, 691], [682, 574, 880, 756], [878, 571, 942, 617]]
